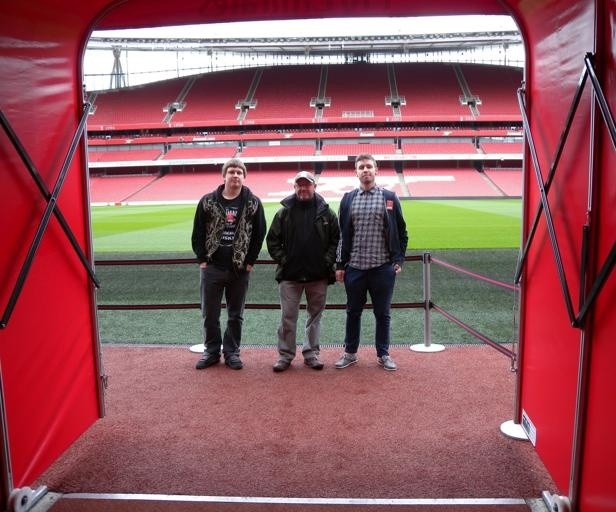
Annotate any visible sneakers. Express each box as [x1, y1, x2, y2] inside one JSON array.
[[196, 351, 221, 370], [377, 355, 397, 370], [224, 354, 243, 370], [273, 355, 291, 372], [304, 357, 324, 368], [334, 352, 358, 369]]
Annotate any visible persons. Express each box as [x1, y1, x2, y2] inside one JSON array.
[[267, 171, 339, 373], [334, 154, 410, 370], [190, 159, 267, 370]]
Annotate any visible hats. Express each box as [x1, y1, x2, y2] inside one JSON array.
[[294, 171, 316, 184]]
